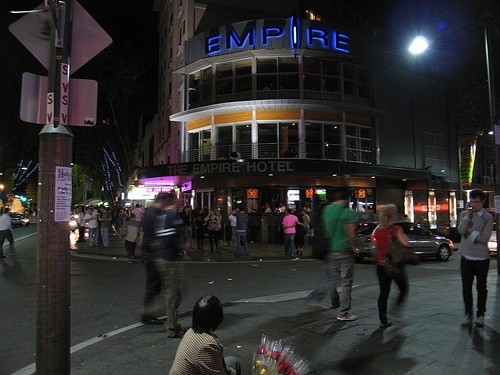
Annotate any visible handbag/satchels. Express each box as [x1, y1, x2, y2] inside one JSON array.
[[387, 231, 415, 266]]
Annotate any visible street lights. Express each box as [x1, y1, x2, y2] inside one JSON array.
[[407, 13, 500, 277]]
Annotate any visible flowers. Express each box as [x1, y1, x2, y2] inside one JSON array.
[[248, 333, 311, 375]]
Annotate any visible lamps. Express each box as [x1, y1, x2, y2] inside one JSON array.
[[230, 151, 244, 163]]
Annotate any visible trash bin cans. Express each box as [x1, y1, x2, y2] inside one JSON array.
[[247, 212, 270, 242]]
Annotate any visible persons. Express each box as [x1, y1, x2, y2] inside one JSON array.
[[303, 202, 311, 212], [265, 204, 271, 212], [0, 208, 14, 259], [304, 189, 361, 321], [140, 190, 191, 338], [372, 203, 411, 328], [169, 296, 241, 375], [457, 190, 493, 327], [76, 204, 145, 264], [278, 202, 286, 213], [181, 206, 250, 256], [281, 208, 311, 257]]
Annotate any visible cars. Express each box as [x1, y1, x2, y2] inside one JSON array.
[[9, 212, 30, 227], [486, 223, 497, 254], [352, 220, 454, 262]]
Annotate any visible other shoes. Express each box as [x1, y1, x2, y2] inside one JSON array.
[[197, 243, 224, 252], [300, 250, 303, 256], [129, 256, 133, 263], [379, 321, 392, 328], [337, 315, 357, 321], [167, 324, 188, 338], [475, 316, 484, 324], [0, 255, 6, 258], [296, 251, 300, 255], [461, 314, 472, 326], [290, 253, 294, 256], [141, 314, 164, 324]]
